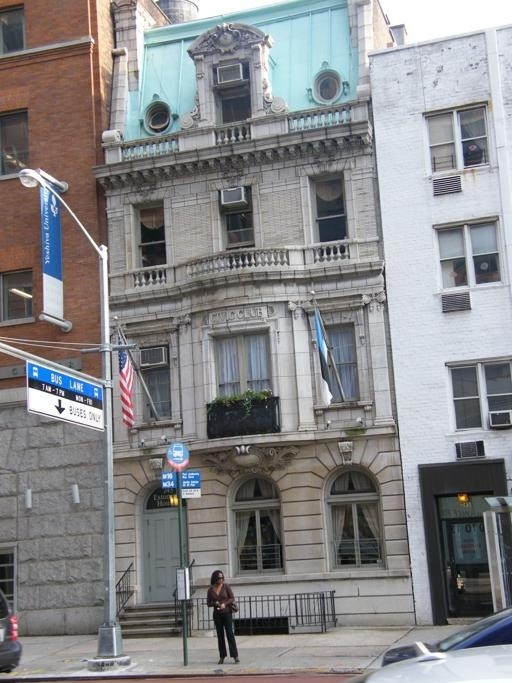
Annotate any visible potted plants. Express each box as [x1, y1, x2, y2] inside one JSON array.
[[206, 388, 282, 440]]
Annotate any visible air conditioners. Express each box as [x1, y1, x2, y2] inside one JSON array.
[[139, 347, 169, 367], [221, 187, 248, 207], [489, 409, 512, 427], [217, 63, 244, 84]]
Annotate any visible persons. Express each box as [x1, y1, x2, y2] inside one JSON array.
[[207, 570, 241, 666]]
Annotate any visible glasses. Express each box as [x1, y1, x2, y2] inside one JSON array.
[[218, 577, 223, 580]]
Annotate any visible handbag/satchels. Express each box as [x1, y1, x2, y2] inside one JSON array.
[[232, 603, 239, 613]]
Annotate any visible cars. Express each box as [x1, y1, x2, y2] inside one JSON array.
[[347, 607, 512, 683]]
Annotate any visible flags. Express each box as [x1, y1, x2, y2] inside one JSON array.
[[314, 303, 334, 408], [117, 329, 135, 431]]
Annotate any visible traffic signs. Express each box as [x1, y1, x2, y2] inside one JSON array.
[[26, 359, 105, 434]]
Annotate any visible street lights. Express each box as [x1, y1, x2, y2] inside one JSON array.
[[16, 167, 132, 673]]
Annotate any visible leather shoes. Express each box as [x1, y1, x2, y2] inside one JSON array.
[[218, 657, 225, 664], [234, 656, 240, 662]]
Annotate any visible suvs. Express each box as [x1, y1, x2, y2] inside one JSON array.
[[0, 587, 23, 672]]
